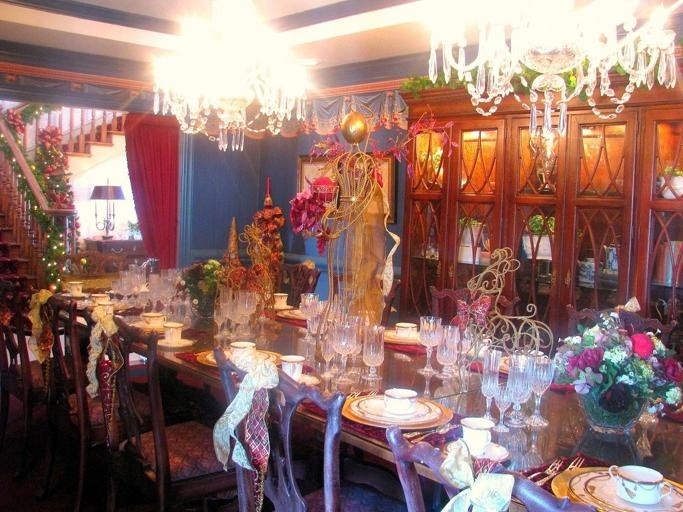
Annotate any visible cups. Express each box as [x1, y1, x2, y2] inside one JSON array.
[[465, 334, 492, 358], [515, 348, 548, 359], [98, 303, 114, 316], [65, 282, 84, 296], [395, 322, 418, 340], [141, 313, 167, 328], [163, 322, 184, 344], [609, 465, 672, 504], [230, 341, 256, 356], [383, 387, 417, 414], [460, 416, 495, 454], [273, 293, 289, 307], [91, 294, 108, 305], [280, 355, 305, 382]]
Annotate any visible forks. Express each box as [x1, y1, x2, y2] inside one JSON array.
[[535, 457, 585, 487], [347, 390, 363, 399], [367, 390, 378, 397], [527, 459, 565, 480]]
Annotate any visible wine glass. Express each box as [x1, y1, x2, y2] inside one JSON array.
[[213, 289, 257, 340], [300, 292, 321, 341], [521, 426, 544, 471], [331, 325, 356, 384], [436, 324, 459, 380], [480, 349, 502, 425], [525, 356, 555, 428], [360, 327, 385, 381], [503, 356, 529, 429], [492, 388, 512, 433], [346, 316, 363, 378], [109, 265, 177, 305], [417, 316, 442, 375], [320, 343, 335, 378]]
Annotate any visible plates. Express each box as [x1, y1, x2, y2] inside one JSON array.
[[270, 306, 293, 310], [285, 310, 304, 321], [551, 466, 683, 512], [61, 292, 89, 298], [442, 439, 510, 464], [196, 348, 283, 367], [384, 330, 422, 344], [342, 395, 454, 430], [294, 374, 322, 387], [157, 339, 193, 348]]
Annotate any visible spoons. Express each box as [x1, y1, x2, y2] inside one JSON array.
[[411, 422, 450, 444]]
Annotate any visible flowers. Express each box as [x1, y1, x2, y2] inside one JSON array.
[[291, 176, 332, 251]]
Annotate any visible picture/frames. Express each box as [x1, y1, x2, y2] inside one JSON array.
[[295, 153, 399, 225]]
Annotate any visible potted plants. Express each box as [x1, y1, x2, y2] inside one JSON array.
[[659, 165, 683, 199], [459, 215, 487, 247], [520, 215, 557, 260]]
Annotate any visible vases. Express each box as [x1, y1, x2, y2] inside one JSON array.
[[314, 198, 386, 360]]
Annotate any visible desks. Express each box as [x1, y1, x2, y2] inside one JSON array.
[[82, 237, 144, 273]]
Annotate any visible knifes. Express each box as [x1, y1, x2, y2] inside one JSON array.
[[402, 424, 459, 439]]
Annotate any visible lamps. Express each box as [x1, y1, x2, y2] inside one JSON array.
[[89, 177, 125, 240], [419, 0, 683, 158], [149, 0, 324, 153]]
